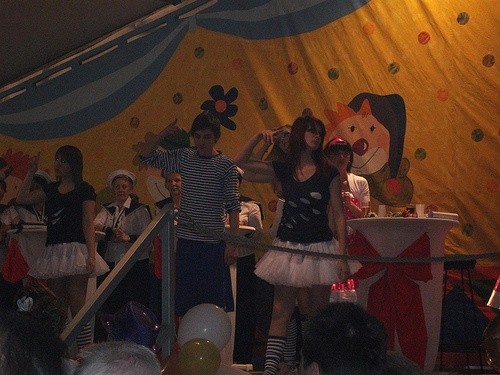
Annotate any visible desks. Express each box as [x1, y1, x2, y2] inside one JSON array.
[[346, 216, 458, 375]]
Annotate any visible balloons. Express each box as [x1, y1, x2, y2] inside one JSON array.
[[109, 301, 251, 375]]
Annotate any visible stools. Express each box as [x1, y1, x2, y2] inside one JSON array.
[[438, 260, 485, 375]]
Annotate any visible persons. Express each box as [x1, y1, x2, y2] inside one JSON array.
[[135, 110, 242, 324], [232, 115, 348, 375], [241, 126, 311, 374], [322, 137, 371, 304], [0, 124, 181, 375], [299, 302, 390, 375], [222, 167, 262, 367]]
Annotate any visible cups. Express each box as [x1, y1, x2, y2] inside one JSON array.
[[407, 204, 416, 214], [378, 204, 386, 217], [416, 204, 425, 216], [359, 201, 370, 216]]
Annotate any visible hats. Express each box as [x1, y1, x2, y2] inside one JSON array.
[[107, 169, 137, 186], [100, 300, 163, 354], [35, 169, 51, 184]]
